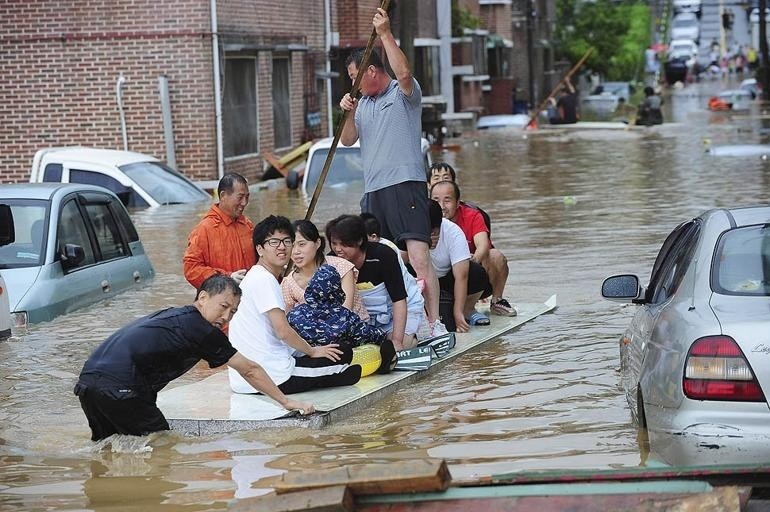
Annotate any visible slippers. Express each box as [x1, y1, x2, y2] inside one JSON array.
[[467, 311, 491, 325]]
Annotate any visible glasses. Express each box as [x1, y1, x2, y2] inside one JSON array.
[[262, 236, 292, 248]]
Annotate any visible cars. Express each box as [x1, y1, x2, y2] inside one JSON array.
[[594, 82, 635, 97], [669, 40, 699, 58], [582, 96, 624, 106], [670, 13, 701, 42], [601, 205, 770, 466]]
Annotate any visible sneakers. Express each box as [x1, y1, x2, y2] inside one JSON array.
[[428, 319, 450, 339], [488, 298, 518, 317]]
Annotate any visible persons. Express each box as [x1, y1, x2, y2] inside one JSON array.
[[547, 77, 578, 125], [285, 266, 389, 348], [586, 69, 613, 96], [427, 163, 491, 230], [227, 215, 362, 394], [358, 213, 398, 254], [616, 97, 635, 112], [279, 221, 370, 327], [74, 273, 315, 442], [709, 43, 758, 79], [399, 198, 471, 332], [185, 173, 258, 337], [635, 88, 664, 125], [722, 9, 734, 28], [430, 181, 517, 317], [326, 214, 417, 352], [339, 8, 449, 337]]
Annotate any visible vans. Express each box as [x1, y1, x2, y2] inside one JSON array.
[[285, 138, 433, 192], [0, 182, 155, 329], [28, 148, 209, 207], [672, 0, 702, 14]]
